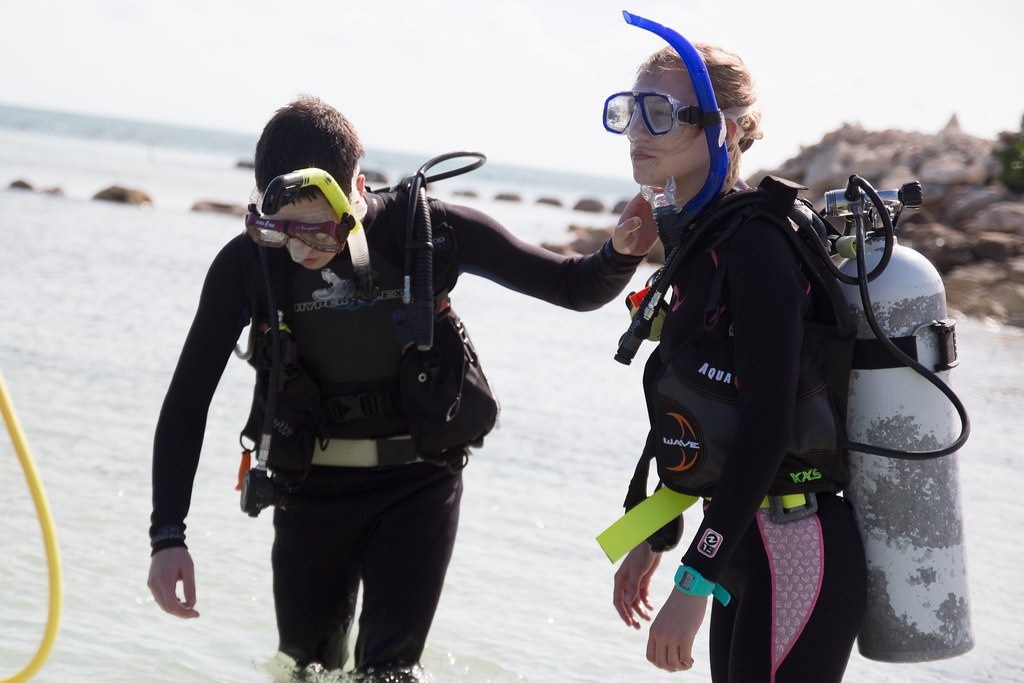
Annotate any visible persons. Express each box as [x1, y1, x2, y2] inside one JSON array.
[[613, 42, 866, 683], [145, 93, 661, 672]]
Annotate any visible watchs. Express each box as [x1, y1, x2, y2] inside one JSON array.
[[674, 565, 732, 607]]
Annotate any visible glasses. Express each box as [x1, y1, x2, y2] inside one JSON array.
[[603, 91, 675, 136], [245, 215, 342, 251]]
